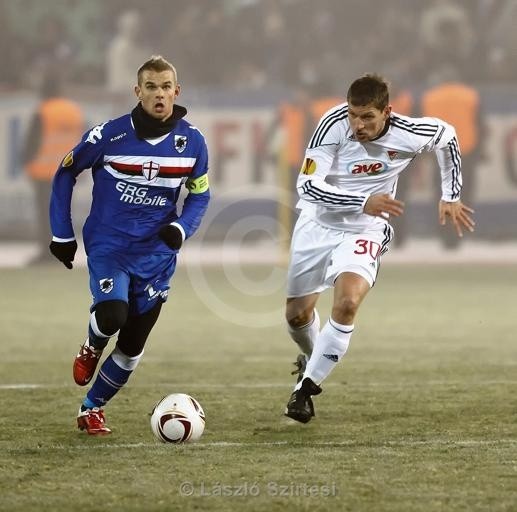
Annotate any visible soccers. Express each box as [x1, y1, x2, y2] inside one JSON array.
[[151, 393, 206, 443]]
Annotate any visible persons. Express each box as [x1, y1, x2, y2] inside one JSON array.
[[284, 71, 475, 424], [252, 62, 482, 258], [48, 55, 212, 435], [14, 70, 84, 269], [101, 8, 153, 105]]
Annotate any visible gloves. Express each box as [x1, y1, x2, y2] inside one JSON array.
[[49, 240, 77, 269], [159, 226, 182, 252]]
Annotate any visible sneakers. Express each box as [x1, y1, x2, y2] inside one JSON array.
[[285, 378, 322, 424], [73, 337, 102, 386], [78, 405, 111, 434], [296, 354, 321, 393]]
